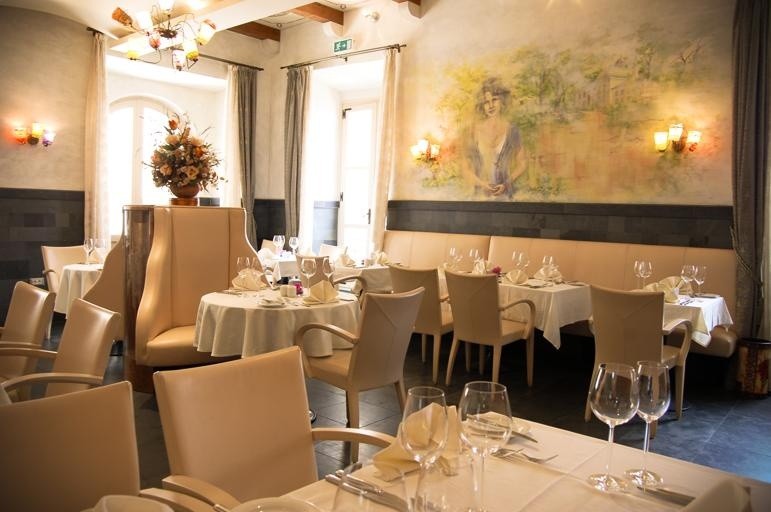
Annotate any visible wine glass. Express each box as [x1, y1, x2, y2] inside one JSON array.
[[457, 381, 513, 512], [272, 235, 282, 253], [251, 257, 265, 297], [453, 248, 463, 271], [512, 251, 529, 270], [95, 239, 106, 263], [633, 260, 645, 291], [289, 236, 298, 255], [401, 387, 450, 512], [682, 264, 697, 299], [300, 258, 317, 297], [448, 247, 456, 263], [83, 239, 93, 264], [281, 235, 285, 251], [639, 262, 652, 291], [693, 265, 707, 295], [468, 248, 478, 262], [543, 256, 556, 265], [322, 257, 336, 281], [586, 361, 641, 495], [624, 359, 671, 486], [236, 256, 250, 299]]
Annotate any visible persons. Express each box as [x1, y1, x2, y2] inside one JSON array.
[[461, 76, 529, 199]]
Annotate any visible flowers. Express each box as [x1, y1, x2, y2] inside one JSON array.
[[141, 109, 229, 192]]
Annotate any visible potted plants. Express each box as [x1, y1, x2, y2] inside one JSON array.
[[730, 225, 770, 401]]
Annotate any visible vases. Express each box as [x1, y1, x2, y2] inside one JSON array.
[[169, 181, 199, 197]]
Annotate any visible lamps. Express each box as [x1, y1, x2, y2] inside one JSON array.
[[15, 122, 56, 148], [112, 0, 216, 71], [410, 140, 440, 165], [654, 124, 700, 153]]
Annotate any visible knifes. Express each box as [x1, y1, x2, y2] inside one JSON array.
[[335, 469, 408, 507], [463, 414, 540, 444], [324, 473, 412, 511]]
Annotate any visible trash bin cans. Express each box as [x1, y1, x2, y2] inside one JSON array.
[[736, 338, 771, 398]]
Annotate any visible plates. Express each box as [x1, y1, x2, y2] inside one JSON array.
[[465, 414, 535, 438], [256, 301, 287, 308], [236, 496, 323, 512]]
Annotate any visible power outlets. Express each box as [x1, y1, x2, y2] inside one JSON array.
[[29, 278, 44, 285]]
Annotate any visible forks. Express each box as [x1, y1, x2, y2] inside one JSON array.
[[521, 452, 559, 464], [490, 447, 523, 458]]
